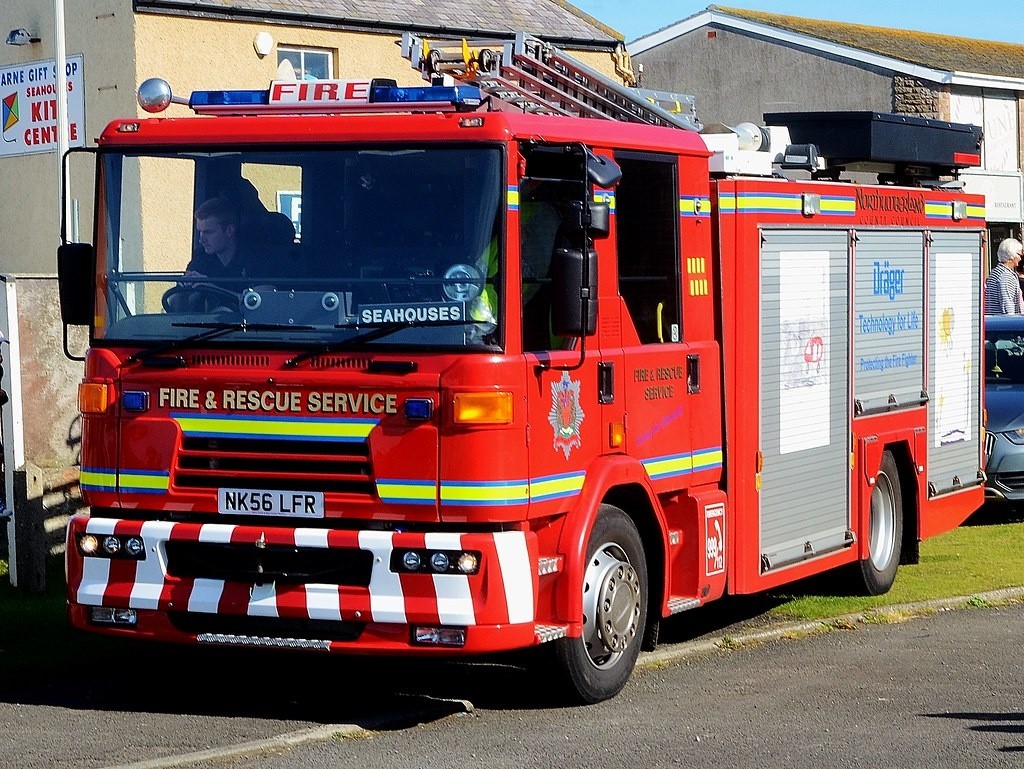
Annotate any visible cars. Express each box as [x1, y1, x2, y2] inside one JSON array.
[[984, 314, 1024, 514]]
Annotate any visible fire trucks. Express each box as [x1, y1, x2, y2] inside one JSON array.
[[53, 26, 988, 708]]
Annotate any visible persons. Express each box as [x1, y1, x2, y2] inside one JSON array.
[[985, 238, 1024, 315], [169, 198, 274, 313]]
